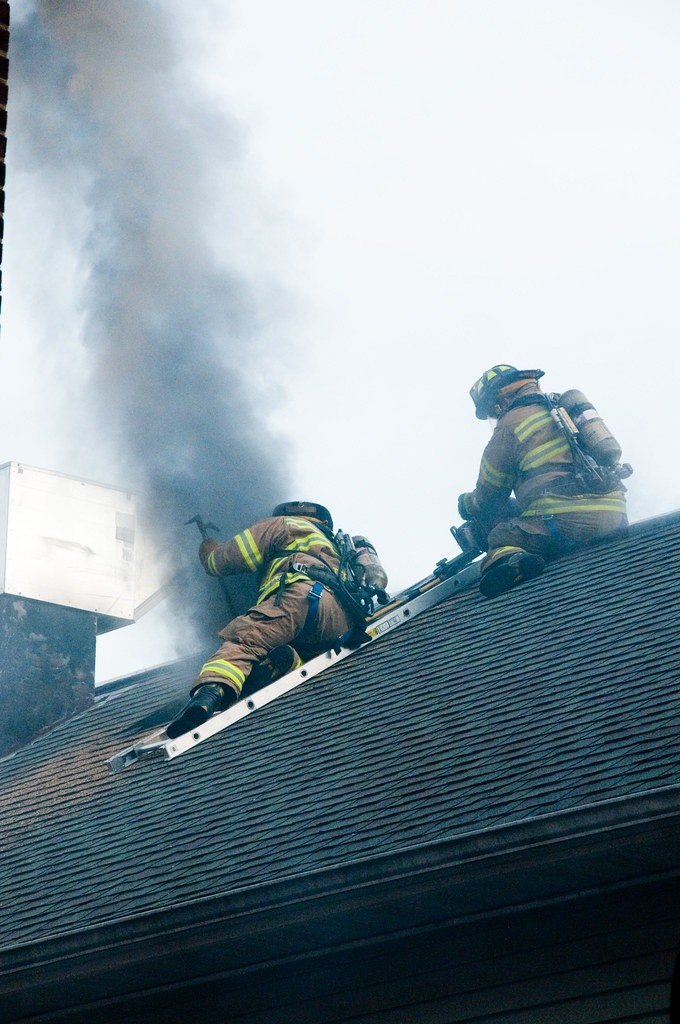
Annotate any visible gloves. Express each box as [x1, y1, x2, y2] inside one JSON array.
[[457, 493, 470, 521]]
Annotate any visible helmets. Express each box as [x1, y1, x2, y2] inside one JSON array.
[[272, 501, 333, 532], [469, 361, 545, 421]]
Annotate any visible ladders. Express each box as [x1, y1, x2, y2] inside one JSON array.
[[101, 547, 488, 775]]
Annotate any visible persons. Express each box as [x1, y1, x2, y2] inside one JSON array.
[[160, 501, 362, 739], [458, 364, 629, 598]]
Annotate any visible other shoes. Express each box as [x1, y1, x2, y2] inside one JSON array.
[[237, 642, 305, 710], [479, 551, 545, 601], [166, 685, 221, 741]]
